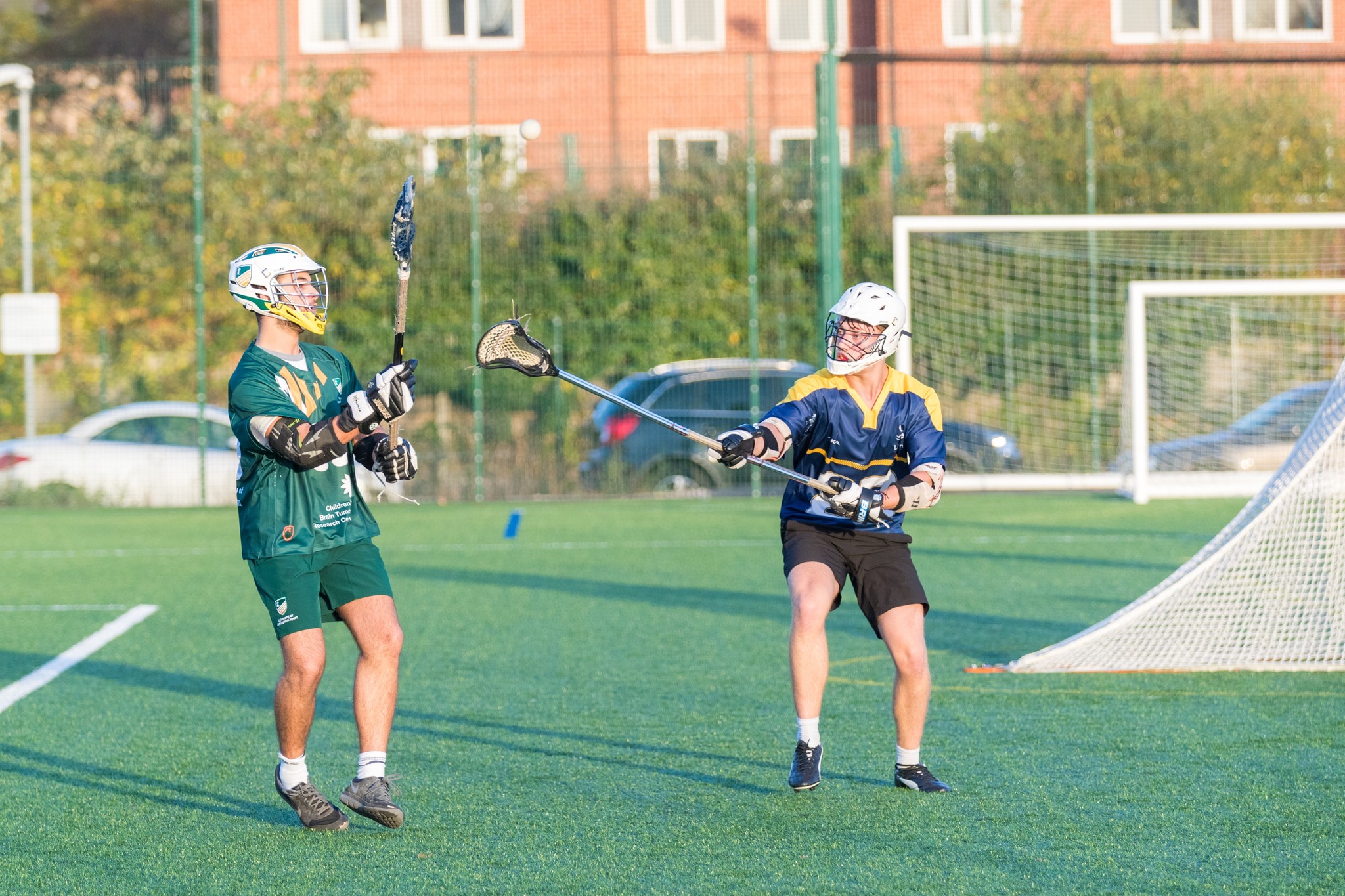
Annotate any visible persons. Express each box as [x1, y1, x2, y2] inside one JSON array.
[[227, 242, 420, 833], [706, 282, 950, 794]]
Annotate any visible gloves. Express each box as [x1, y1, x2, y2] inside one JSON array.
[[707, 423, 765, 470], [351, 431, 419, 482], [822, 476, 892, 531], [338, 359, 419, 435]]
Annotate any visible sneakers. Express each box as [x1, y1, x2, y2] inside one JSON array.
[[339, 774, 405, 828], [274, 763, 350, 832], [788, 739, 823, 793], [893, 762, 950, 794]]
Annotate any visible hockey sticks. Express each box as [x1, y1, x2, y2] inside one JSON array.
[[391, 174, 417, 451], [476, 318, 841, 494]]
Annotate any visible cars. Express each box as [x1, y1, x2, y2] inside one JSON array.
[[1112, 379, 1345, 473], [0, 400, 405, 510]]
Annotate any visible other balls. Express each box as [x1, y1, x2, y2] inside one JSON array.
[[520, 118, 541, 140]]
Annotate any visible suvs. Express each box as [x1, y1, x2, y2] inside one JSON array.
[[576, 356, 1021, 499]]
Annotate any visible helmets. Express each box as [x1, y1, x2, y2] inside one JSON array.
[[824, 282, 907, 375], [227, 243, 327, 334]]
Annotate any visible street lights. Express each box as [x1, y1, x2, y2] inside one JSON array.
[[0, 62, 39, 439]]
